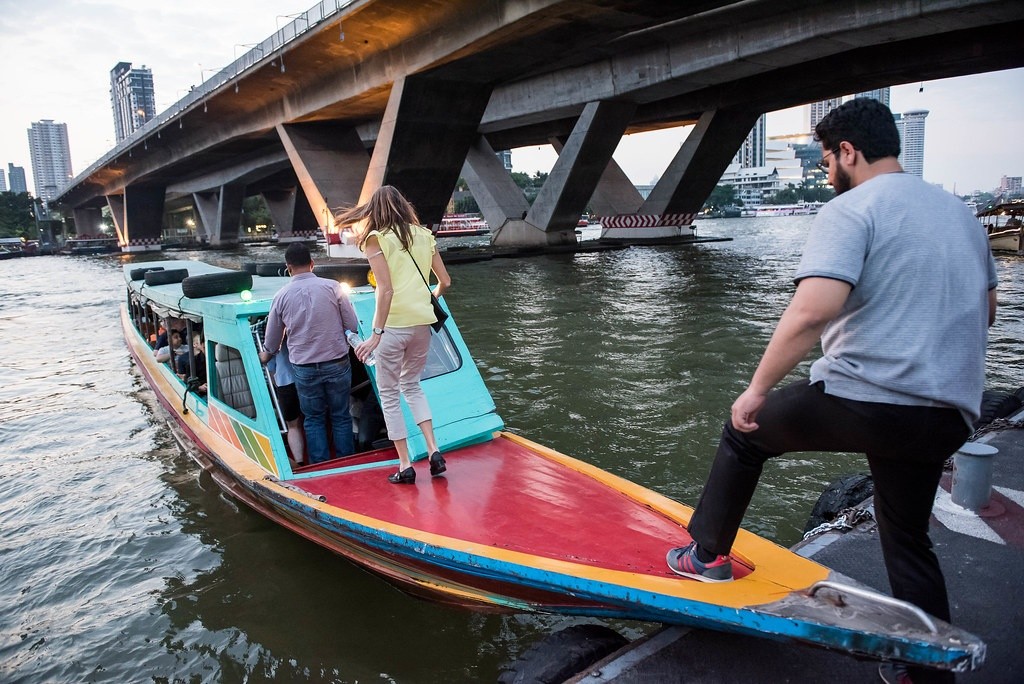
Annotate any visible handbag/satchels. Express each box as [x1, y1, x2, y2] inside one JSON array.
[[430, 294, 448, 333]]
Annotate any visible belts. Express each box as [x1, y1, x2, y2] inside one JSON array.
[[295, 354, 348, 367]]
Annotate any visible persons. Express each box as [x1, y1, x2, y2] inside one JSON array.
[[257, 243, 387, 465], [152, 316, 207, 400], [665, 97, 999, 684], [1007, 214, 1018, 224], [335, 186, 451, 485]]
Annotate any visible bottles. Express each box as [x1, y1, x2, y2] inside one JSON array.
[[345, 330, 376, 367]]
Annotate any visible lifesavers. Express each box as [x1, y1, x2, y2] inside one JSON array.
[[313, 264, 371, 287], [130, 266, 164, 280], [240, 262, 289, 276], [144, 268, 189, 286], [181, 270, 253, 299]]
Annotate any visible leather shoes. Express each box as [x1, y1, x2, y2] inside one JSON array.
[[388, 466, 416, 484], [429, 451, 446, 475]]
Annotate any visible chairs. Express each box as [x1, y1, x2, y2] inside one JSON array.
[[213, 343, 257, 421]]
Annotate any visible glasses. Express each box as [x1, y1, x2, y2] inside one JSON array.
[[815, 145, 860, 174]]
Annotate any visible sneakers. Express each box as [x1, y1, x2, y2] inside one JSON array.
[[666, 541, 734, 583], [879, 662, 913, 684]]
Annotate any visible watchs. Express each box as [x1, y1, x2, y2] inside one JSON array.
[[371, 327, 384, 334]]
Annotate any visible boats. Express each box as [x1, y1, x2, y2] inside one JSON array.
[[117, 255, 990, 675], [434, 216, 491, 237], [756, 204, 818, 217], [976, 202, 1024, 254], [575, 219, 591, 229], [59, 231, 120, 255]]
